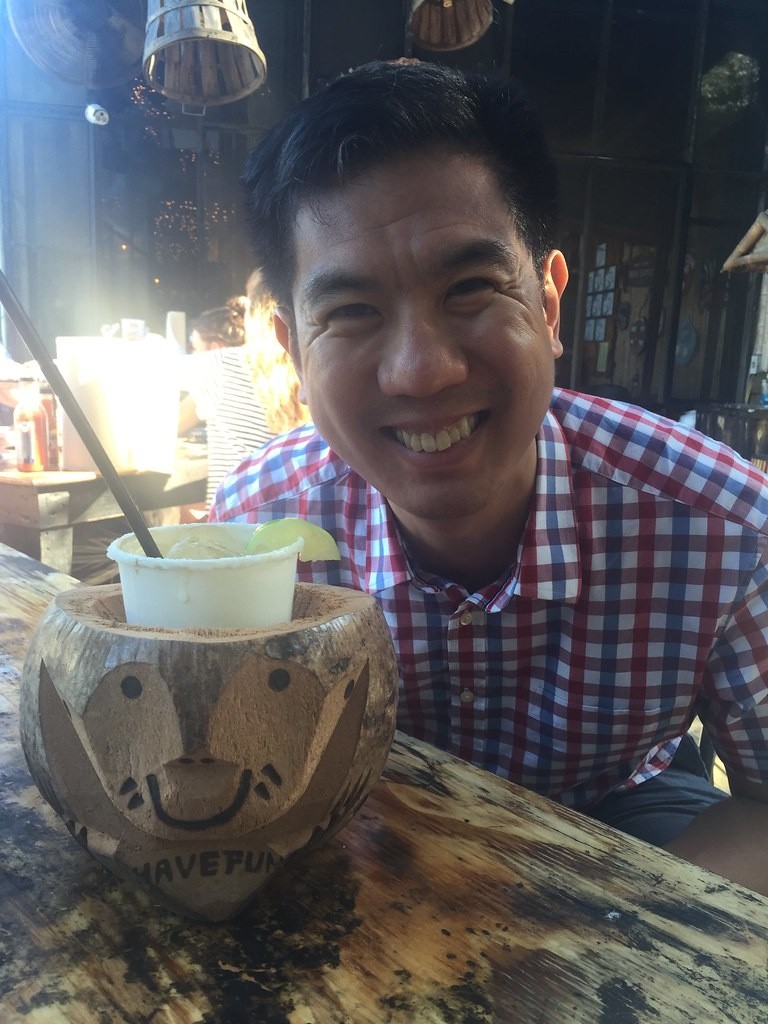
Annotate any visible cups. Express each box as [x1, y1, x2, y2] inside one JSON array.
[[120, 318, 145, 341], [110, 522, 304, 630]]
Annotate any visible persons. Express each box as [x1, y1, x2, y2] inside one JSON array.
[[146, 266, 311, 523], [207, 59, 767, 893]]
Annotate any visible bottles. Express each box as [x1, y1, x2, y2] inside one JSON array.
[[1, 376, 57, 472]]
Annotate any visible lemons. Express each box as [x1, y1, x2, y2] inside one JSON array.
[[246, 517, 342, 561]]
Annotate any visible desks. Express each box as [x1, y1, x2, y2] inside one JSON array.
[[0, 540, 768, 1024], [0, 457, 207, 573]]
